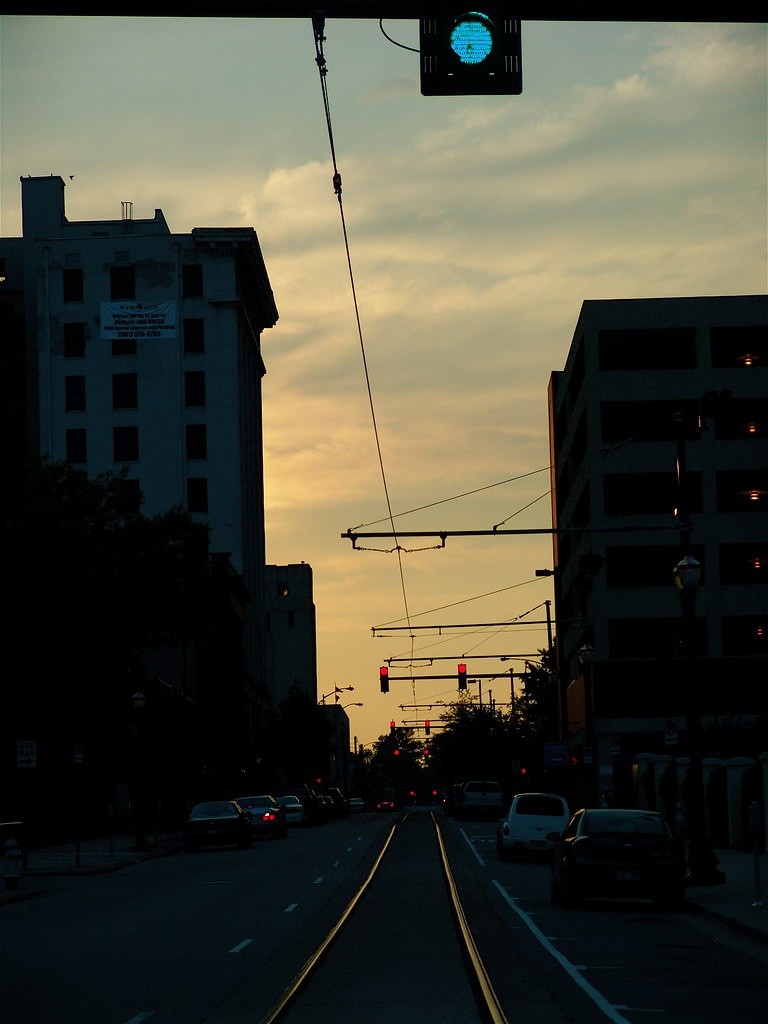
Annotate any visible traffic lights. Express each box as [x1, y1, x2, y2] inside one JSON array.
[[390, 721, 397, 736], [424, 720, 432, 736], [393, 749, 402, 757], [570, 746, 583, 769], [380, 666, 389, 693], [423, 749, 430, 755], [420, 18, 523, 96], [520, 767, 531, 786], [458, 663, 468, 689]]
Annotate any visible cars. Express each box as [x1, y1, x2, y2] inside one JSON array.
[[183, 801, 254, 852], [232, 796, 289, 839], [546, 807, 687, 908], [499, 792, 569, 855], [297, 788, 366, 825], [276, 795, 305, 823], [460, 780, 506, 814], [376, 801, 396, 813]]
